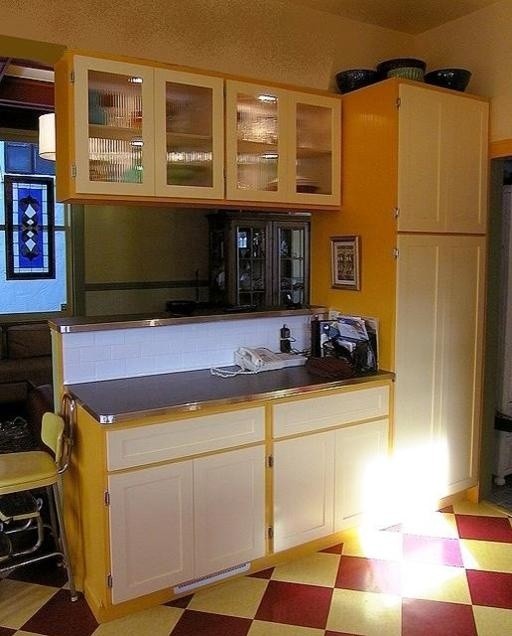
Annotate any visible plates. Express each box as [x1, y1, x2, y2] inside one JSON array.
[[268, 178, 321, 193]]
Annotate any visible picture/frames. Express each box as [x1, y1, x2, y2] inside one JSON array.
[[328, 234, 362, 292]]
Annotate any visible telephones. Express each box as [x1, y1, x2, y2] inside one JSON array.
[[234, 346, 283, 373], [280, 324, 292, 353]]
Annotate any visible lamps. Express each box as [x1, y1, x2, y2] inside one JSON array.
[[37, 109, 59, 164]]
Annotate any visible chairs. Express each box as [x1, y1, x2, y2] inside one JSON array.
[[1, 395, 79, 604]]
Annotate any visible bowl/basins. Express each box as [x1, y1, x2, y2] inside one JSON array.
[[336, 69, 377, 93], [424, 68, 471, 92], [377, 58, 425, 81]]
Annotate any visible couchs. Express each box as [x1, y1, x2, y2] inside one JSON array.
[[0, 319, 54, 403]]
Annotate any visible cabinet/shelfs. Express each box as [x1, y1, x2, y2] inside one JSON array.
[[224, 73, 342, 213], [310, 235, 487, 519], [270, 378, 392, 568], [314, 77, 489, 235], [209, 213, 309, 309], [54, 49, 225, 206], [56, 391, 270, 623]]
